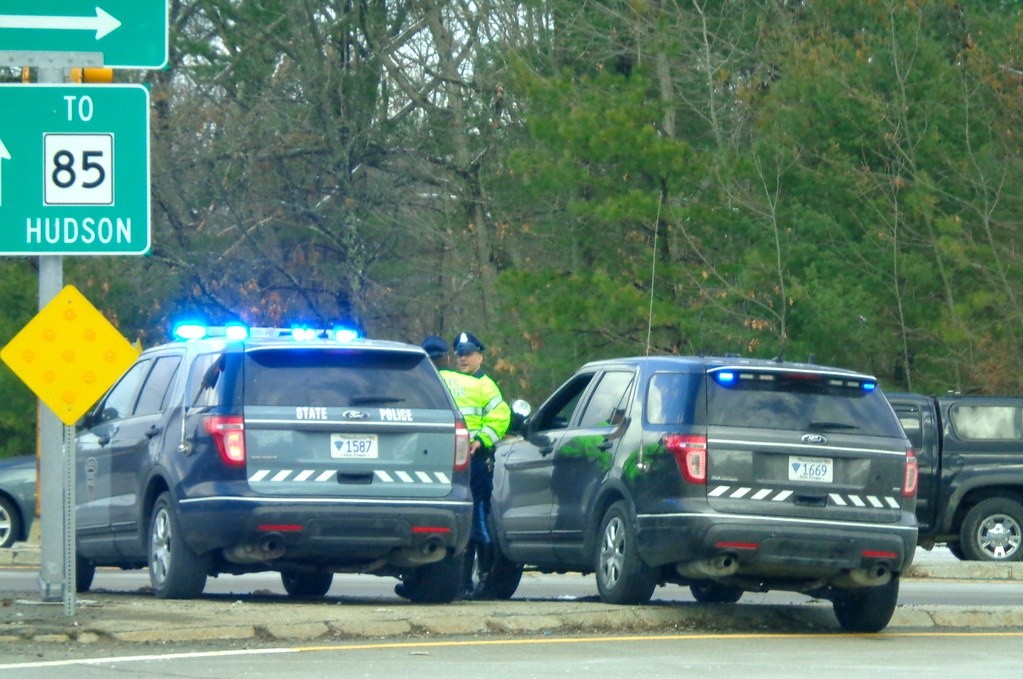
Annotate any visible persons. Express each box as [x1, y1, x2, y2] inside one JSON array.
[[395, 336, 510, 600], [450, 333, 503, 601]]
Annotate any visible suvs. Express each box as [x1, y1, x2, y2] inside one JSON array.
[[74, 317, 475, 605], [466, 354, 918, 634]]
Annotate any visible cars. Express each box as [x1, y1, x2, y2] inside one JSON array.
[[0, 454, 40, 550]]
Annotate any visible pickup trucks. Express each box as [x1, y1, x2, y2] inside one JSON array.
[[884, 393, 1023, 564]]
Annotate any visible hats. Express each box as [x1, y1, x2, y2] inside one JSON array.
[[421, 335, 448, 358], [453, 332, 485, 352]]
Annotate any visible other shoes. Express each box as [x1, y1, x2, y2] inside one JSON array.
[[480, 571, 488, 581], [463, 588, 474, 596]]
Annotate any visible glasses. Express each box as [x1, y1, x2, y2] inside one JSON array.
[[456, 351, 472, 356]]
